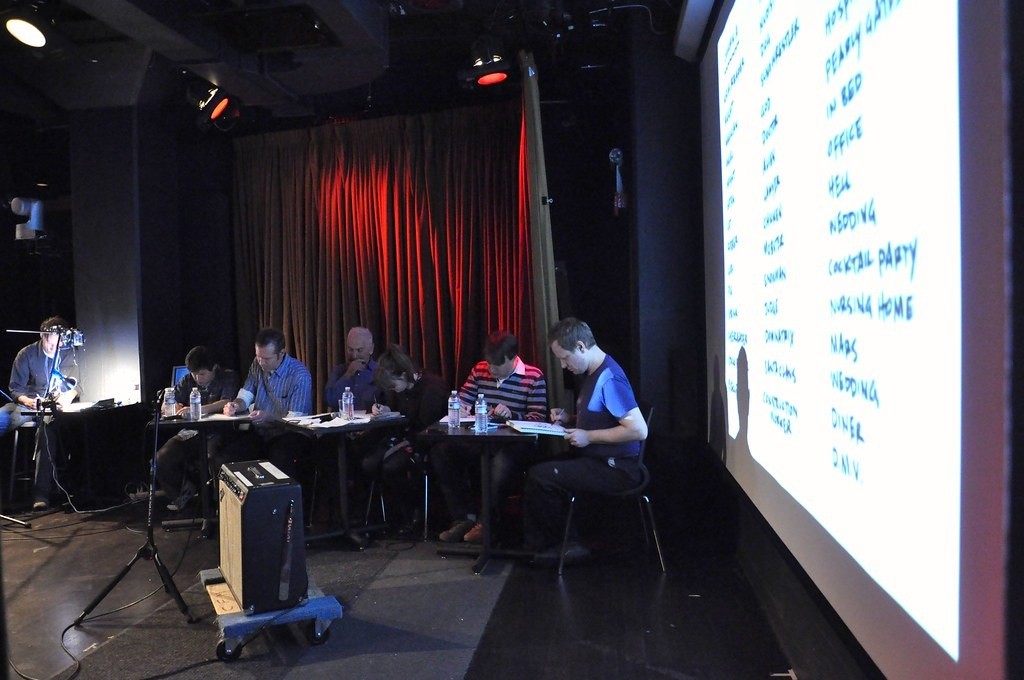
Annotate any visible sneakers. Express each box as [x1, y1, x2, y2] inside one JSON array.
[[464, 522, 485, 542], [439, 516, 477, 542]]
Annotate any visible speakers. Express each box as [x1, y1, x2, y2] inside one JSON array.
[[217, 459, 309, 616]]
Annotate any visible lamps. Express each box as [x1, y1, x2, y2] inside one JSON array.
[[181, 78, 233, 119], [5, 0, 57, 48], [469, 35, 510, 84]]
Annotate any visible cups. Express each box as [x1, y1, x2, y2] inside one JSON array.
[[338, 399, 343, 418]]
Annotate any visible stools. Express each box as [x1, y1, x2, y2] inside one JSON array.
[[10, 421, 33, 502]]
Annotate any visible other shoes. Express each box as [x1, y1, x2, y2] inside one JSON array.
[[519, 537, 589, 566], [398, 518, 424, 539], [167, 480, 196, 510], [33, 493, 49, 508], [375, 514, 393, 537]]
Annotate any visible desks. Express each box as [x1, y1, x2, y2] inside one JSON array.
[[415, 417, 543, 573], [282, 411, 409, 551], [145, 411, 270, 537]]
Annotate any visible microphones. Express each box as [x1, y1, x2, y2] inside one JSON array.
[[312, 413, 335, 422]]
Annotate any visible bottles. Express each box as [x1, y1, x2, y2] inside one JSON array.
[[475, 394, 487, 434], [448, 390, 460, 429], [164, 388, 176, 421], [189, 387, 201, 421], [342, 386, 354, 420]]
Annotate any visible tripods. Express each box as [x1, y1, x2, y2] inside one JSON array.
[[74, 389, 193, 628]]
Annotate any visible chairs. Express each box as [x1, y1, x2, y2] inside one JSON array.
[[554, 407, 666, 576]]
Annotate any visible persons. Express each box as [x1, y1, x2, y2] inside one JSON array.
[[147, 345, 242, 512], [0, 315, 85, 509], [211, 327, 313, 485], [528, 317, 649, 561], [322, 326, 377, 547], [361, 342, 448, 529], [428, 331, 547, 541]]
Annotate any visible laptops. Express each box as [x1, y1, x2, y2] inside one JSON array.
[[171, 366, 190, 387]]
